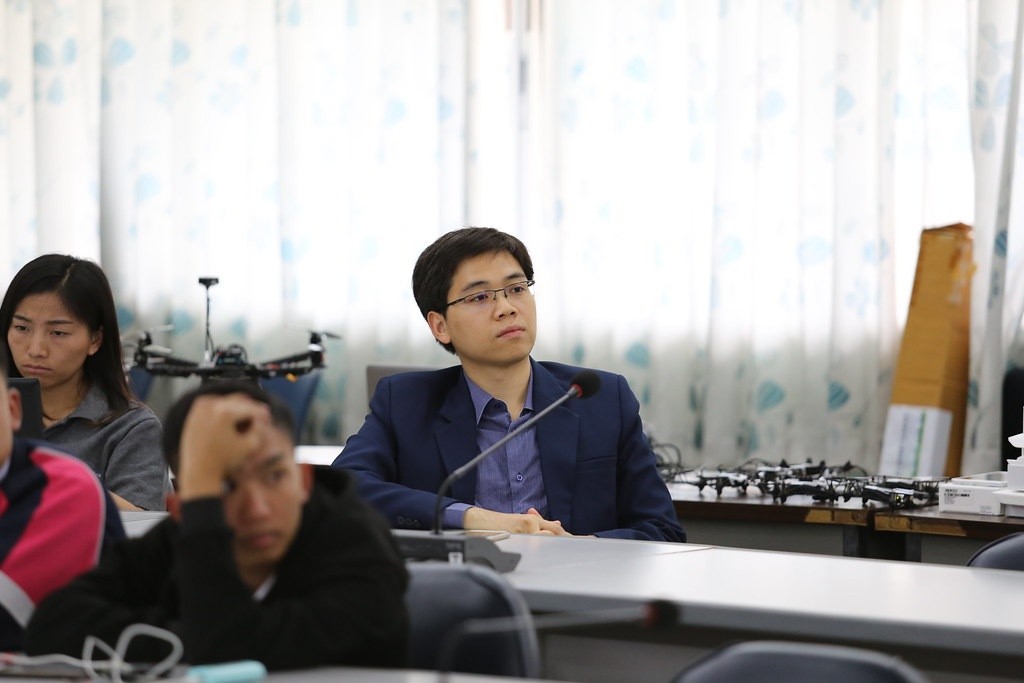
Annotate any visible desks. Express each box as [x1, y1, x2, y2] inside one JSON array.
[[122, 510, 1024, 683], [296, 442, 1024, 572]]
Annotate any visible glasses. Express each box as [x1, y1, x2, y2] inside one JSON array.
[[434, 281, 535, 307]]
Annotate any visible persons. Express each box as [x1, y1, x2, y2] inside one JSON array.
[[330, 227, 687, 542], [0, 254, 166, 514], [22, 384, 414, 672], [0, 355, 105, 654]]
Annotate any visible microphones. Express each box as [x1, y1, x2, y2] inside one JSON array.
[[390, 371, 601, 573]]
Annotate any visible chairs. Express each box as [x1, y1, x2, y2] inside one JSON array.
[[965, 531, 1024, 572], [669, 639, 927, 683], [404, 567, 542, 682]]
